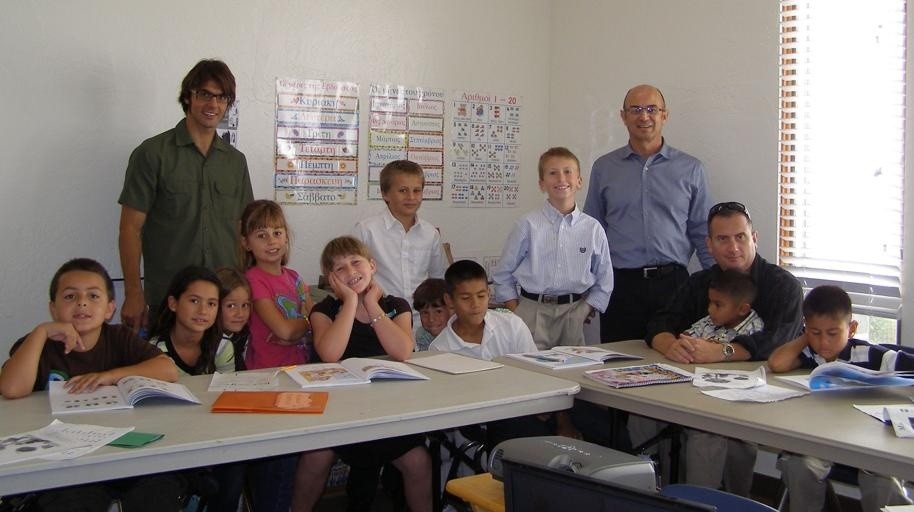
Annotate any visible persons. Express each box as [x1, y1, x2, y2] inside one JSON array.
[[145, 200, 556, 511], [117, 56, 258, 340], [350, 159, 449, 352], [628, 204, 803, 512], [1, 256, 180, 401], [678, 265, 764, 342], [491, 147, 616, 445], [768, 284, 914, 510], [584, 85, 714, 339]]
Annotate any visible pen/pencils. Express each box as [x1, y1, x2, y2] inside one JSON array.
[[523, 355, 564, 362]]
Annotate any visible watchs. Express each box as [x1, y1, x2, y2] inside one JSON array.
[[719, 341, 736, 362]]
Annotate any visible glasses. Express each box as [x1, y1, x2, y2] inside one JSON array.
[[624, 107, 666, 115], [190, 88, 231, 104], [709, 201, 751, 221]]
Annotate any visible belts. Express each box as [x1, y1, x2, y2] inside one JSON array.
[[643, 265, 673, 280], [520, 287, 582, 305]]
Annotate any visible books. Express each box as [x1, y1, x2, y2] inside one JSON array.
[[774, 359, 914, 396], [505, 341, 648, 373], [44, 378, 203, 418], [584, 361, 694, 391], [852, 403, 914, 439]]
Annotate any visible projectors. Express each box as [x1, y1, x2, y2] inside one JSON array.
[[489, 435, 657, 493]]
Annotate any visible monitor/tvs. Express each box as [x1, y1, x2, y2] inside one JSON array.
[[501, 459, 718, 512]]
